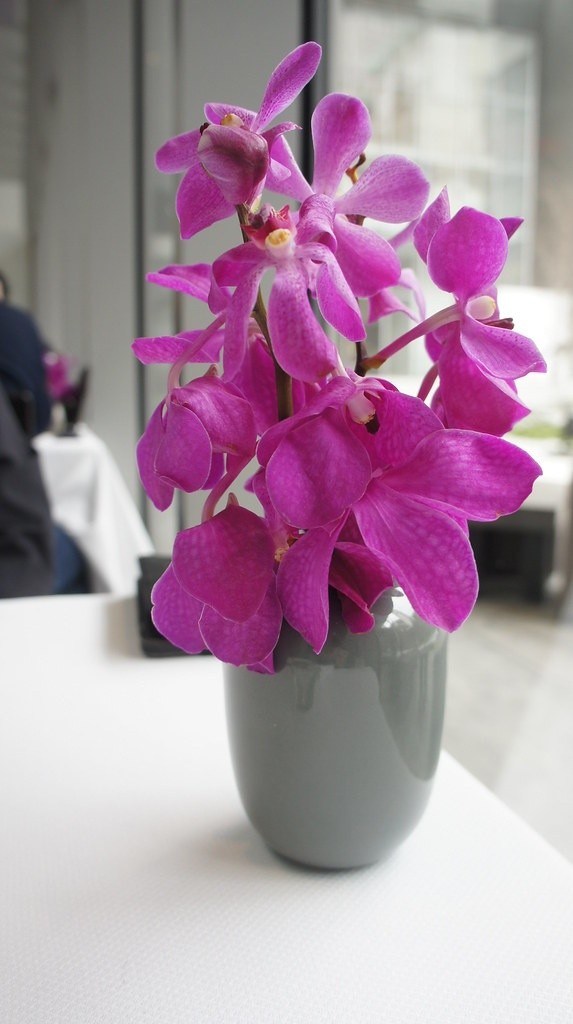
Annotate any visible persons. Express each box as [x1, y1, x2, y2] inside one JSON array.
[[0, 272, 89, 598]]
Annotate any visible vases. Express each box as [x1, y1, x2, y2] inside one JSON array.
[[52, 402, 67, 433], [225, 579, 449, 868]]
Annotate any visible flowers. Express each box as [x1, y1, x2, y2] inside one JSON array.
[[130, 42, 548, 675], [42, 351, 73, 402]]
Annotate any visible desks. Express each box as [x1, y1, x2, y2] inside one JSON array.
[[32, 423, 153, 594], [0, 593, 573, 1024]]
